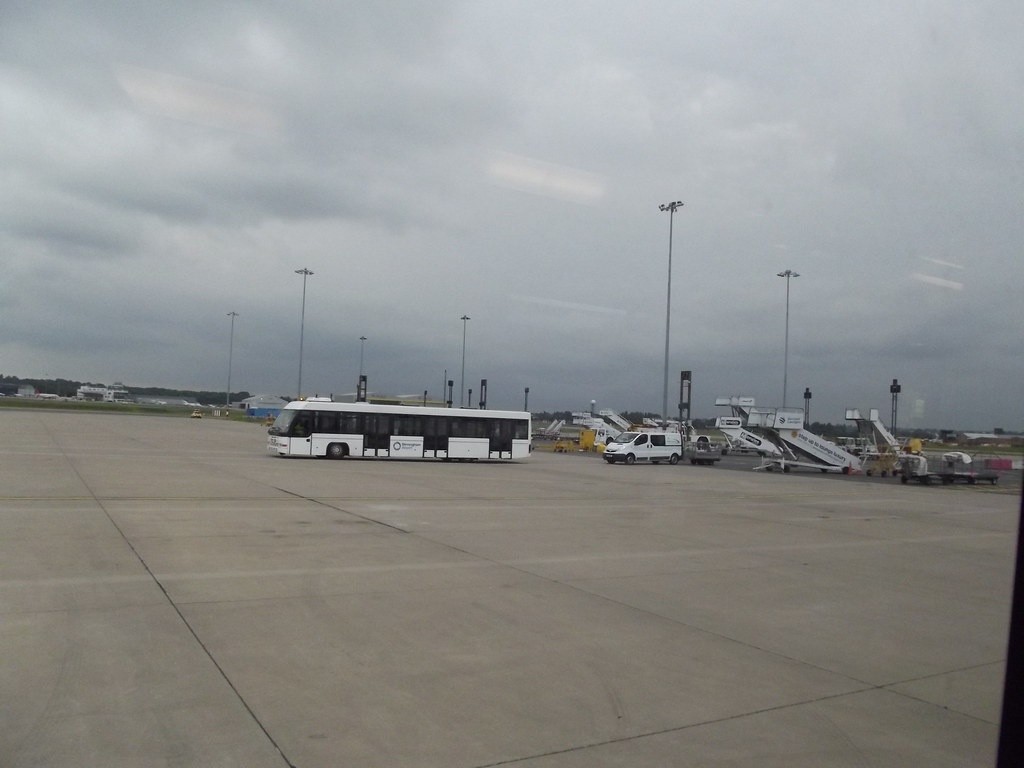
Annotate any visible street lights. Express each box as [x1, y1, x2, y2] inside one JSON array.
[[225, 310, 239, 409], [358, 335, 368, 376], [776, 269, 800, 409], [657, 200, 684, 431], [294, 267, 314, 404], [460, 315, 471, 408]]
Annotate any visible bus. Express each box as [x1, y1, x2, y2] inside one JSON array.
[[267, 397, 532, 463]]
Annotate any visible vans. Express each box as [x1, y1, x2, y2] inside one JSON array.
[[603, 432, 681, 465]]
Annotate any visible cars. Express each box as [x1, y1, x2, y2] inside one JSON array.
[[191, 409, 202, 418]]
